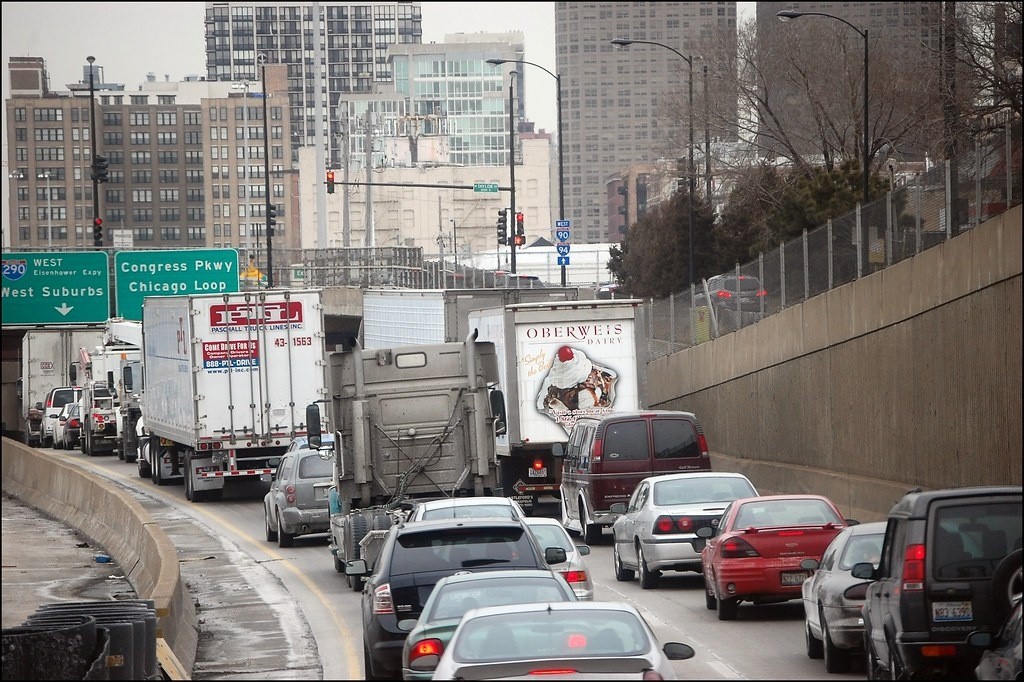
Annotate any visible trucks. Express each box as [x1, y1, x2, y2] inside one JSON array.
[[70, 317, 143, 462], [357, 286, 580, 349], [550, 408, 712, 545], [467, 297, 643, 513], [17, 326, 106, 447]]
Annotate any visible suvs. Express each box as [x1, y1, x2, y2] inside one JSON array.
[[343, 516, 567, 681], [852, 478, 1024, 681], [692, 272, 767, 321]]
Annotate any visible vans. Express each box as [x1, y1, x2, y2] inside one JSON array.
[[36, 385, 84, 448]]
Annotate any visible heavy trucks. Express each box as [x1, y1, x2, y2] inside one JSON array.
[[122, 289, 324, 501], [306, 328, 505, 591]]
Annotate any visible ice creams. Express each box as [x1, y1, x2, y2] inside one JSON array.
[[536, 347, 619, 434]]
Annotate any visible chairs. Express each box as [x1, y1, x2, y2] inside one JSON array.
[[850, 542, 880, 567], [936, 532, 964, 566]]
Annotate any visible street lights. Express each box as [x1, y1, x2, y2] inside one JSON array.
[[38, 171, 59, 250], [233, 80, 253, 261], [8, 170, 25, 250], [86, 56, 99, 248], [508, 71, 516, 275], [486, 58, 567, 285], [612, 38, 696, 308], [776, 10, 869, 273]]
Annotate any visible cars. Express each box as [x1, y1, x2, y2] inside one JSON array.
[[610, 474, 761, 588], [396, 567, 696, 682], [348, 267, 548, 304], [50, 400, 80, 452], [696, 493, 997, 674], [965, 593, 1024, 682], [402, 495, 593, 601]]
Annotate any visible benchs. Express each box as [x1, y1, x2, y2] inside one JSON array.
[[478, 629, 624, 659]]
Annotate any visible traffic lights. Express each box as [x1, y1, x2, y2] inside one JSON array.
[[92, 154, 108, 183], [498, 210, 506, 246], [515, 236, 525, 245], [94, 218, 102, 246], [326, 172, 334, 194], [268, 204, 277, 229], [516, 213, 524, 236]]
